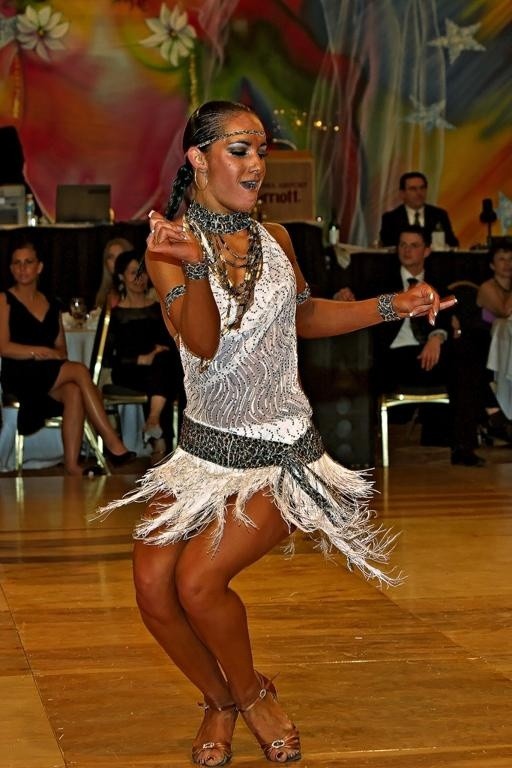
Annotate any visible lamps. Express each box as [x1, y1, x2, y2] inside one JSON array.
[[478, 197, 498, 252]]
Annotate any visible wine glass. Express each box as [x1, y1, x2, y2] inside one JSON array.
[[70, 297, 88, 329]]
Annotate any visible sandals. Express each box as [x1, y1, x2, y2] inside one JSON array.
[[191, 703, 238, 766], [239, 669, 302, 763]]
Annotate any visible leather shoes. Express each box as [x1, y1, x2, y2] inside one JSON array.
[[103, 442, 138, 467], [478, 410, 511, 443], [451, 453, 486, 468], [65, 468, 99, 476]]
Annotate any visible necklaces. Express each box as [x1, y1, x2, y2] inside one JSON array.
[[183, 201, 264, 330]]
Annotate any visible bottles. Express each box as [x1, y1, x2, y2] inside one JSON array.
[[329, 211, 341, 248], [26, 193, 36, 227]]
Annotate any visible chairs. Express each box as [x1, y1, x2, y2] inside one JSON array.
[[376, 388, 451, 468], [0, 401, 113, 478], [103, 303, 179, 452], [446, 280, 481, 332]]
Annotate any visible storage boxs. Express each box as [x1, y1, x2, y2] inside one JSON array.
[[55, 184, 112, 225], [252, 151, 317, 226]]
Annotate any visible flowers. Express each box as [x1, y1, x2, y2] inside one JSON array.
[[0, 4, 72, 63], [137, 1, 198, 68]]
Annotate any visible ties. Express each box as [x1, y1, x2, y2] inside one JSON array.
[[407, 278, 426, 344], [414, 212, 421, 227]]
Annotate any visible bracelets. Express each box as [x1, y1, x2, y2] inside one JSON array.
[[184, 261, 209, 280], [378, 293, 403, 323]]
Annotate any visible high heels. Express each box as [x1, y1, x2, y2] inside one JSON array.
[[142, 427, 162, 447]]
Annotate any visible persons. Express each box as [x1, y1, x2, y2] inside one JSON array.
[[379, 172, 458, 247], [84, 100, 458, 768], [0, 237, 185, 484], [477, 234, 512, 422], [333, 226, 512, 466]]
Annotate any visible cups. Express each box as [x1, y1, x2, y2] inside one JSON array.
[[430, 231, 446, 249]]
